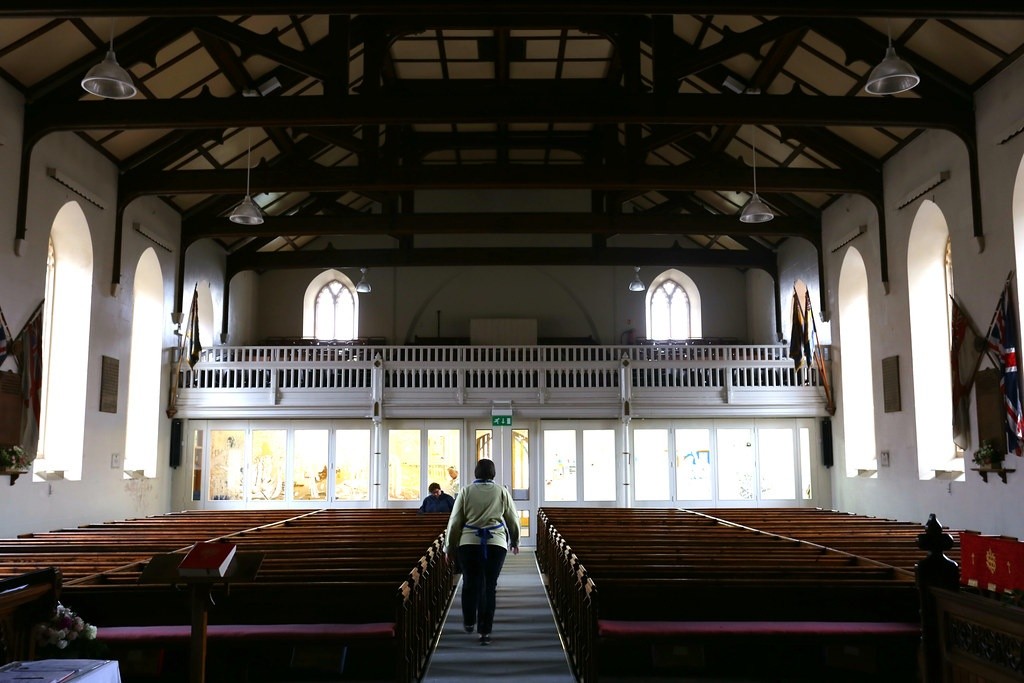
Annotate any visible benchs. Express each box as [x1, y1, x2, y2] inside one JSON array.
[[534, 506, 1023, 683], [0, 509, 467, 682]]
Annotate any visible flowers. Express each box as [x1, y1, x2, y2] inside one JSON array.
[[0, 446, 34, 471], [36, 603, 98, 650]]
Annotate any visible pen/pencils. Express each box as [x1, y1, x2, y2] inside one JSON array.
[[11, 677, 44, 679]]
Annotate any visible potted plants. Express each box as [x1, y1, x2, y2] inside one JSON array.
[[974, 442, 1005, 470]]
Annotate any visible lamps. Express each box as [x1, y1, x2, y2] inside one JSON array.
[[354, 266, 372, 293], [81, 15, 137, 100], [741, 124, 775, 225], [627, 266, 644, 293], [229, 134, 266, 227], [864, 20, 920, 98]]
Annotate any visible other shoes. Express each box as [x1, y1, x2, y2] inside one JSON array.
[[464, 624, 474, 633], [479, 636, 492, 645]]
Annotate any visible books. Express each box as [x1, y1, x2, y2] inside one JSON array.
[[0, 671, 75, 683]]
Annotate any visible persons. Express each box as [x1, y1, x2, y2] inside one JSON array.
[[419, 483, 455, 512], [441, 466, 459, 492], [443, 459, 520, 645]]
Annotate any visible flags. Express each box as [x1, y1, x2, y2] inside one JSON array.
[[0, 310, 12, 366], [789, 296, 805, 372], [950, 301, 982, 451], [804, 291, 815, 371], [986, 287, 1023, 457], [21, 303, 45, 465], [188, 293, 202, 371]]
